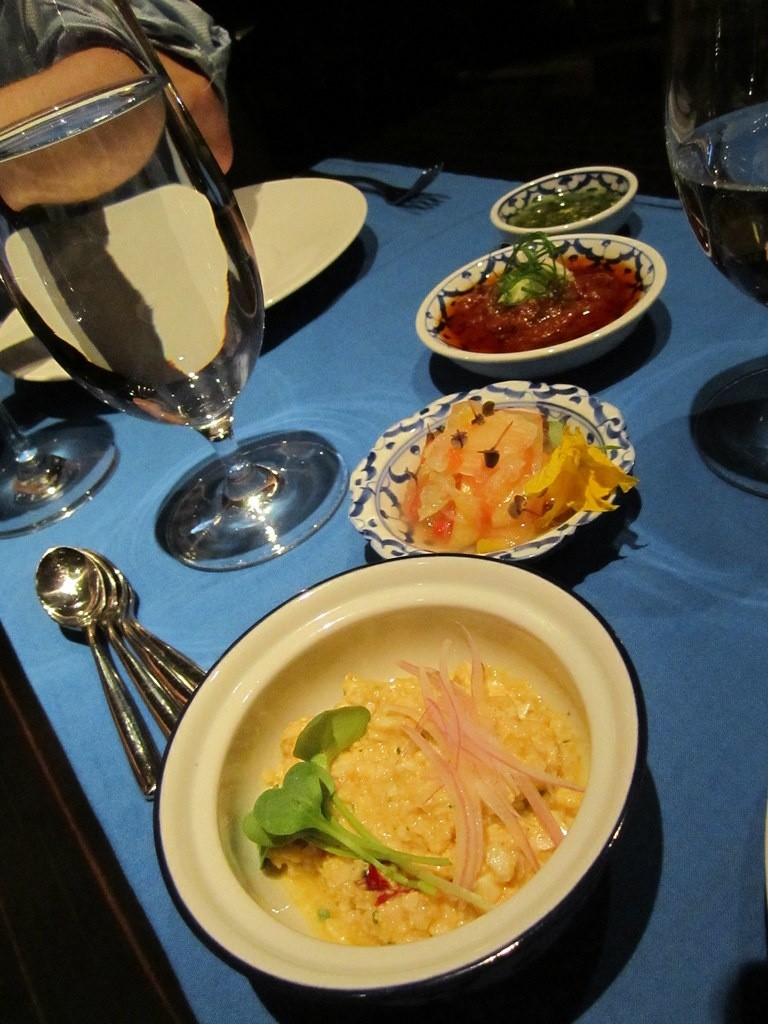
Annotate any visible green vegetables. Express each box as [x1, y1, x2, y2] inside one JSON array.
[[510, 188, 619, 227], [492, 230, 569, 304], [242, 704, 482, 905]]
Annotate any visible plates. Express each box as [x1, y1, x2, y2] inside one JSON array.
[[0, 177, 368, 383], [347, 379, 636, 561]]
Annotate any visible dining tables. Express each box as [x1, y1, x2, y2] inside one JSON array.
[[0, 156, 768, 1024]]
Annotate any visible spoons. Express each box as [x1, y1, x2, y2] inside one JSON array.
[[35, 548, 209, 802]]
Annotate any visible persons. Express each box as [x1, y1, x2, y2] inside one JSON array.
[[0, 0, 235, 176]]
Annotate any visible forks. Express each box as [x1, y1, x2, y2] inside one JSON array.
[[295, 161, 446, 205]]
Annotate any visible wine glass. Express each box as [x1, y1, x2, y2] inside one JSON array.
[[0, 381, 118, 537], [665, 0, 768, 499], [0, 0, 352, 573]]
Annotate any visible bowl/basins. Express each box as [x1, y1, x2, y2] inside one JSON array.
[[416, 233, 668, 380], [489, 165, 639, 247], [152, 553, 648, 1005]]
